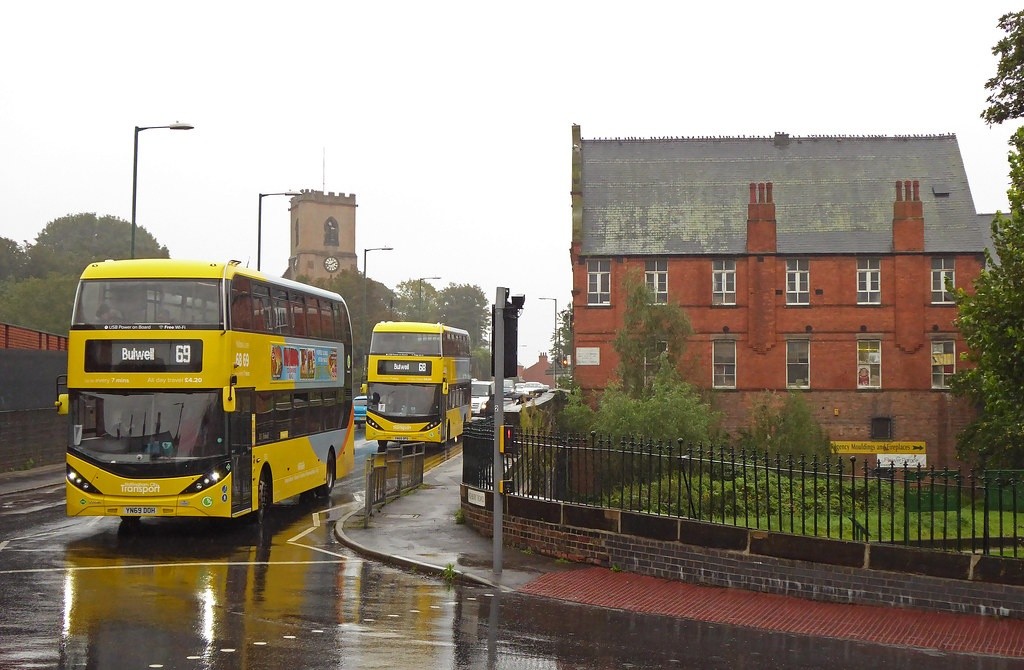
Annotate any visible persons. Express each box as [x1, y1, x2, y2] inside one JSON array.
[[486, 394, 494, 416], [96, 290, 124, 321], [517, 395, 524, 405]]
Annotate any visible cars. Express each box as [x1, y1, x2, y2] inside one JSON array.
[[353, 396, 372, 429], [504, 380, 516, 399], [514, 382, 525, 398], [523, 382, 543, 401]]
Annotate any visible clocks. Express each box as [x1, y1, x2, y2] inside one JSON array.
[[324, 256, 340, 272]]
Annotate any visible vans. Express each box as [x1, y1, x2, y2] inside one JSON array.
[[472, 381, 495, 417]]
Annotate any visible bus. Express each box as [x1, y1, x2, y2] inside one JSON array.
[[55, 259, 355, 527], [360, 321, 472, 448]]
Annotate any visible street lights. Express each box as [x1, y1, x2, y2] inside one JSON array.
[[362, 247, 393, 314], [539, 298, 557, 389], [418, 277, 441, 322], [130, 123, 194, 260], [256, 190, 302, 272]]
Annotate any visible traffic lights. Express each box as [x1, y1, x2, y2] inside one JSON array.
[[500, 425, 514, 454]]
